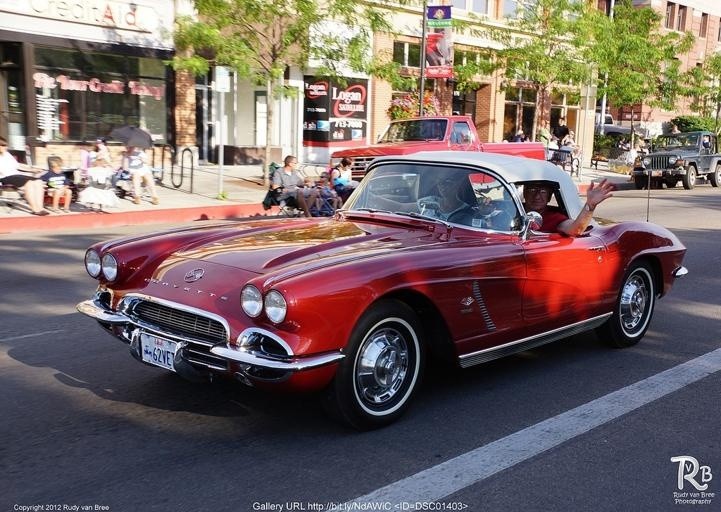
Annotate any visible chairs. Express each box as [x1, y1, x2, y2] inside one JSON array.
[[265, 163, 355, 217]]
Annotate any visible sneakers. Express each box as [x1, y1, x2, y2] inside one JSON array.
[[133, 197, 159, 205], [33, 209, 48, 216]]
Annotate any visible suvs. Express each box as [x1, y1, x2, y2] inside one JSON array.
[[631, 128, 721, 189]]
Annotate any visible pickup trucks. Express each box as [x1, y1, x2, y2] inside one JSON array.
[[328, 112, 548, 193], [592, 111, 648, 147]]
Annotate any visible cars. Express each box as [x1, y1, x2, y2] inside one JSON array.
[[72, 149, 689, 431]]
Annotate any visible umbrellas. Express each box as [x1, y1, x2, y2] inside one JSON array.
[[108, 125, 155, 151]]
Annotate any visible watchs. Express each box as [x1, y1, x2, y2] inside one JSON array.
[[585, 202, 597, 213]]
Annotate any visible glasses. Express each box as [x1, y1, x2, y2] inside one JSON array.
[[525, 186, 550, 196], [432, 178, 457, 188]]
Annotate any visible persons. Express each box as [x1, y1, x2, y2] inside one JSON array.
[[671, 124, 682, 146], [477, 176, 616, 238], [273, 156, 318, 219], [92, 136, 113, 166], [541, 117, 650, 168], [38, 156, 78, 214], [0, 136, 52, 217], [366, 169, 469, 221], [319, 171, 343, 212], [121, 144, 161, 206], [702, 135, 711, 148], [331, 156, 359, 195]]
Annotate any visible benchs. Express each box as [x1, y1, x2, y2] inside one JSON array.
[[547, 148, 580, 177], [591, 147, 646, 174]]
